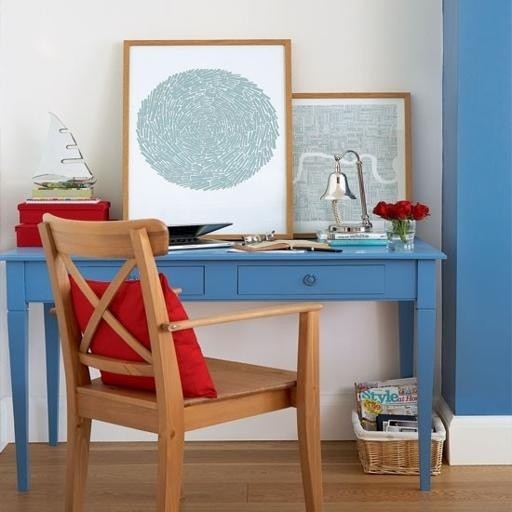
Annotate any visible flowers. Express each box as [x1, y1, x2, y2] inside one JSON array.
[[371, 200, 431, 242]]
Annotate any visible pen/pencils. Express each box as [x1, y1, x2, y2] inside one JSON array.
[[293, 247, 343, 253]]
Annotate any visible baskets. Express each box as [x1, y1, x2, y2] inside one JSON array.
[[352, 407, 447, 476]]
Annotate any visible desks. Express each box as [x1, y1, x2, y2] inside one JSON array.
[[0, 246, 448, 493]]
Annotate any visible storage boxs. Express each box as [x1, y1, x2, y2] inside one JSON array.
[[15, 224, 44, 247], [17, 201, 110, 224]]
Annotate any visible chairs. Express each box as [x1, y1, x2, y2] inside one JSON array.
[[36, 212, 328, 511]]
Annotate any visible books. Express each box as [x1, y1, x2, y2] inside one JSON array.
[[236, 235, 330, 253], [325, 239, 389, 247], [354, 377, 433, 438], [328, 231, 388, 240]]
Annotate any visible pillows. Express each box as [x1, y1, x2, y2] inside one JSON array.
[[66, 272, 217, 400]]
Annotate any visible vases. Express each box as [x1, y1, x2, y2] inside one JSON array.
[[384, 220, 416, 241]]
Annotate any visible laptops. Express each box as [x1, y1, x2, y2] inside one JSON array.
[[167, 223, 233, 250]]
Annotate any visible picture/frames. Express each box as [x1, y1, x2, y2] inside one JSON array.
[[121, 37, 295, 241], [289, 90, 411, 240]]
